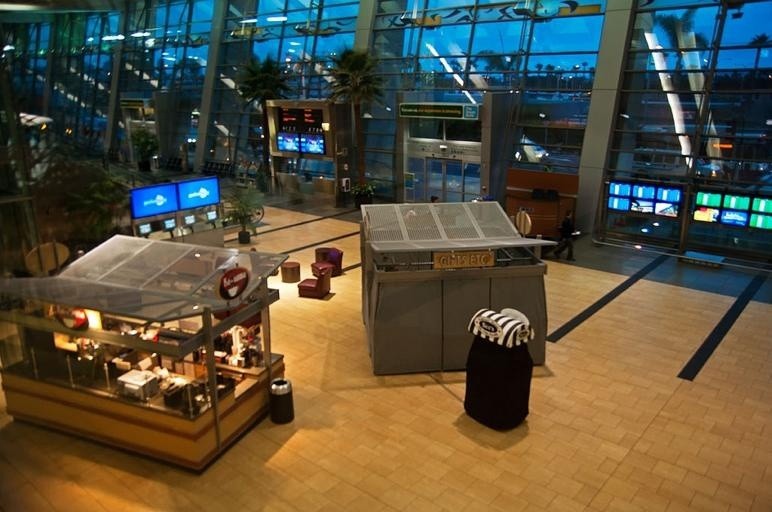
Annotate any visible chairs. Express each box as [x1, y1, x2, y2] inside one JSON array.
[[248, 247, 344, 299]]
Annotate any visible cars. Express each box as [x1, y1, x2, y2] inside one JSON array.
[[514, 135, 549, 164], [727, 160, 772, 187]]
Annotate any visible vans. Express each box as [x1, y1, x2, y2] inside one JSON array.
[[631, 148, 722, 182]]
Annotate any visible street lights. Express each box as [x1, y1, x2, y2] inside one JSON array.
[[556, 64, 583, 97]]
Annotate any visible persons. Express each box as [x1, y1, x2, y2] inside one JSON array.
[[552, 208, 577, 262], [430, 195, 440, 203]]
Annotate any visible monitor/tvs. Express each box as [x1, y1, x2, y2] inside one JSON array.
[[277, 132, 300, 152], [300, 133, 325, 155], [178, 175, 220, 210], [129, 183, 179, 219], [608, 182, 772, 230]]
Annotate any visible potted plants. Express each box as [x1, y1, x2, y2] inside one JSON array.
[[130, 127, 159, 173], [215, 182, 264, 244], [350, 183, 377, 209]]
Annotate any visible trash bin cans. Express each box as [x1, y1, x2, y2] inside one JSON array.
[[334, 186, 346, 209], [268, 377, 294, 425]]
